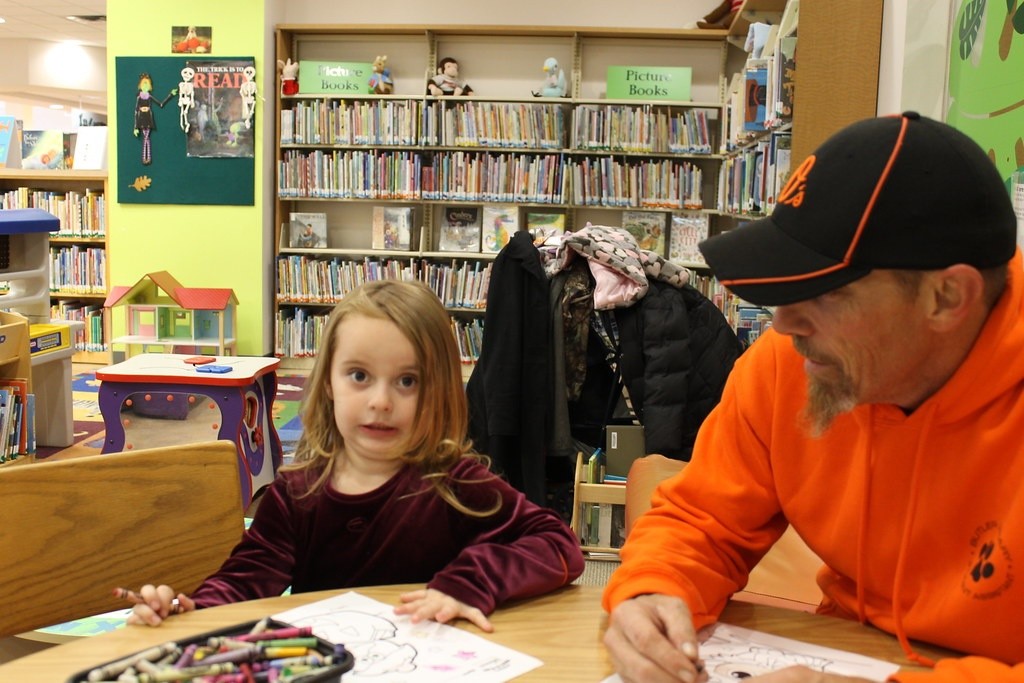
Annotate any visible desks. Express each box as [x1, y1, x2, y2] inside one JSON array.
[[0, 584, 964, 683], [95, 353, 283, 515]]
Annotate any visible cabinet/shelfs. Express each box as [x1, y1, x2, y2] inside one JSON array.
[[272, 0, 883, 371], [0, 168, 113, 367]]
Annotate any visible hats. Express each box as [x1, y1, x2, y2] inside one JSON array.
[[697, 111, 1018, 305]]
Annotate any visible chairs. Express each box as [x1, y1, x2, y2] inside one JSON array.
[[0, 440, 245, 665]]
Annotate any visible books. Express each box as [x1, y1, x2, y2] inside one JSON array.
[[717, 37, 798, 217], [0, 188, 105, 238], [622, 214, 709, 261], [451, 313, 484, 364], [275, 308, 331, 359], [0, 116, 107, 170], [275, 258, 493, 311], [49, 245, 106, 293], [690, 270, 772, 349], [281, 100, 710, 154], [50, 298, 107, 353], [278, 151, 703, 211], [290, 213, 327, 249], [0, 379, 36, 464], [439, 204, 560, 255], [372, 206, 415, 251]]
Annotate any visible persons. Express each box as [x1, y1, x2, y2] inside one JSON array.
[[128, 283, 586, 633], [602, 111, 1024, 683]]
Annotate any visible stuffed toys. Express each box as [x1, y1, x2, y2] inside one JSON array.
[[368, 56, 393, 95], [427, 58, 476, 96], [530, 57, 572, 99], [278, 59, 299, 94]]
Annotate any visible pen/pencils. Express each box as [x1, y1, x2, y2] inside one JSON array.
[[280, 585, 291, 596]]
